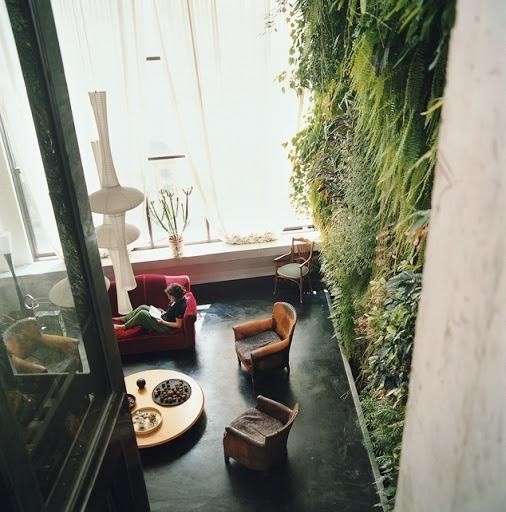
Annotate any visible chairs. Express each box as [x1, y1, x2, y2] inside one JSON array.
[[223, 236, 315, 472], [1, 318, 83, 374]]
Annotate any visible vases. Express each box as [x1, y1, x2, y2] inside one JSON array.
[[168, 236, 183, 260]]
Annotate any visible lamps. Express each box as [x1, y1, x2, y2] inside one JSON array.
[[46, 1, 141, 319]]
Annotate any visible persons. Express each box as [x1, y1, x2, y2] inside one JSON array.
[[111, 283, 186, 334]]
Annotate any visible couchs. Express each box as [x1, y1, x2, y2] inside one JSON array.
[[108, 273, 197, 357]]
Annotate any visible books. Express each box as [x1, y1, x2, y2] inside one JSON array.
[[148, 304, 162, 320]]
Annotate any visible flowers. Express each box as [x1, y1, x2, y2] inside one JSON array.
[[150, 186, 193, 238]]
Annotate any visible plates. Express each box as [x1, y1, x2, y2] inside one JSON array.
[[126, 394, 163, 435]]
